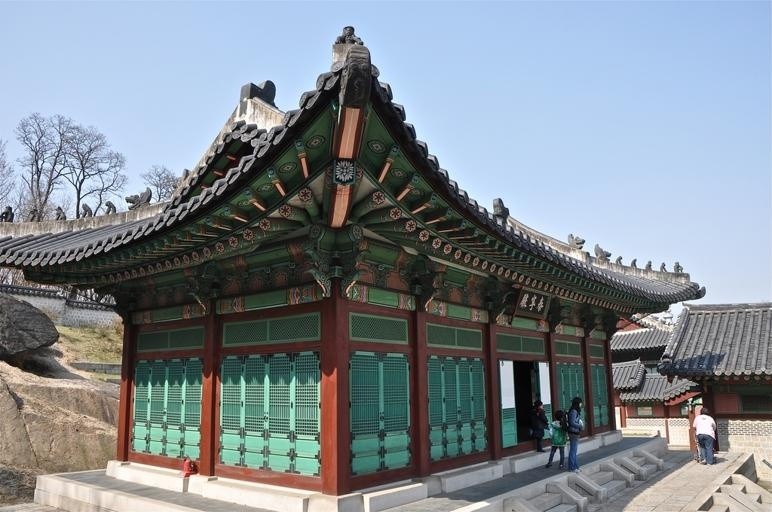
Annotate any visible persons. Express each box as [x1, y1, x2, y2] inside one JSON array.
[[693, 408, 717, 466], [546, 411, 566, 470], [529, 400, 550, 453], [566, 398, 584, 472]]
